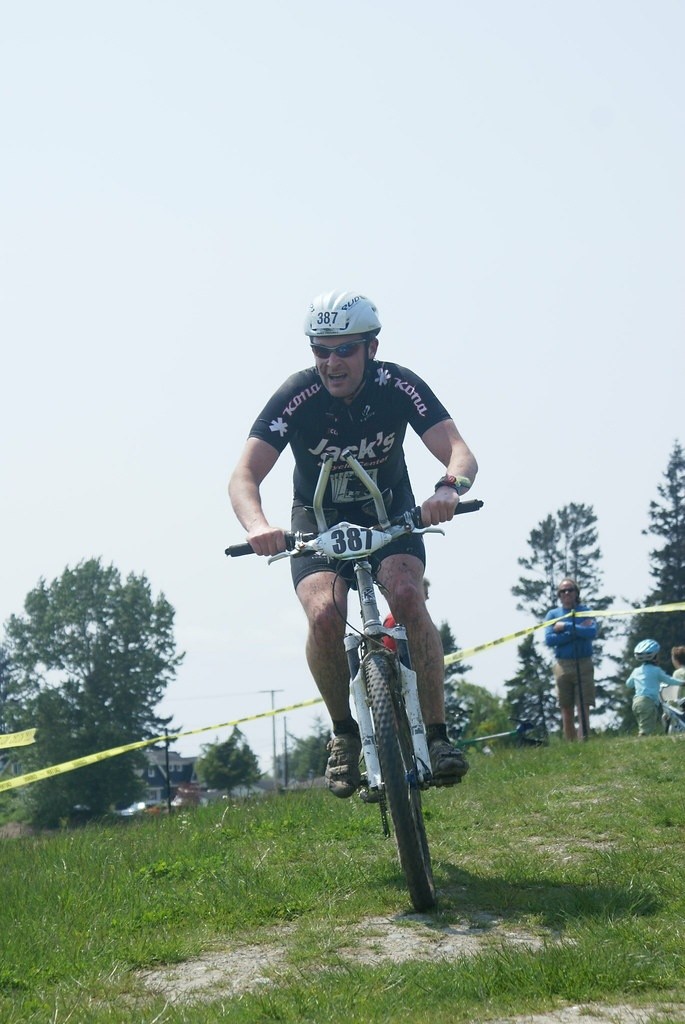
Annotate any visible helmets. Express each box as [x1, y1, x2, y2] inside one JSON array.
[[303, 289, 382, 337], [634, 638, 660, 662]]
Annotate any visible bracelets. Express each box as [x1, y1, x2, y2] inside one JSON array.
[[434, 475, 471, 495]]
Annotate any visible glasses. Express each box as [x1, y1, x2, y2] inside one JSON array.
[[558, 588, 578, 593], [309, 339, 371, 359]]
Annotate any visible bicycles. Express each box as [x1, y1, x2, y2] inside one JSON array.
[[659, 684, 685, 734], [224, 498, 487, 913]]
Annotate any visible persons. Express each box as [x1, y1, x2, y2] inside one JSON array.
[[227, 290, 479, 798], [544, 579, 598, 740], [626, 639, 685, 736], [662, 646, 685, 734]]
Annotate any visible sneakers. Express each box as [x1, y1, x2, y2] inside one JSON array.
[[324, 719, 362, 799], [428, 740, 469, 788]]
